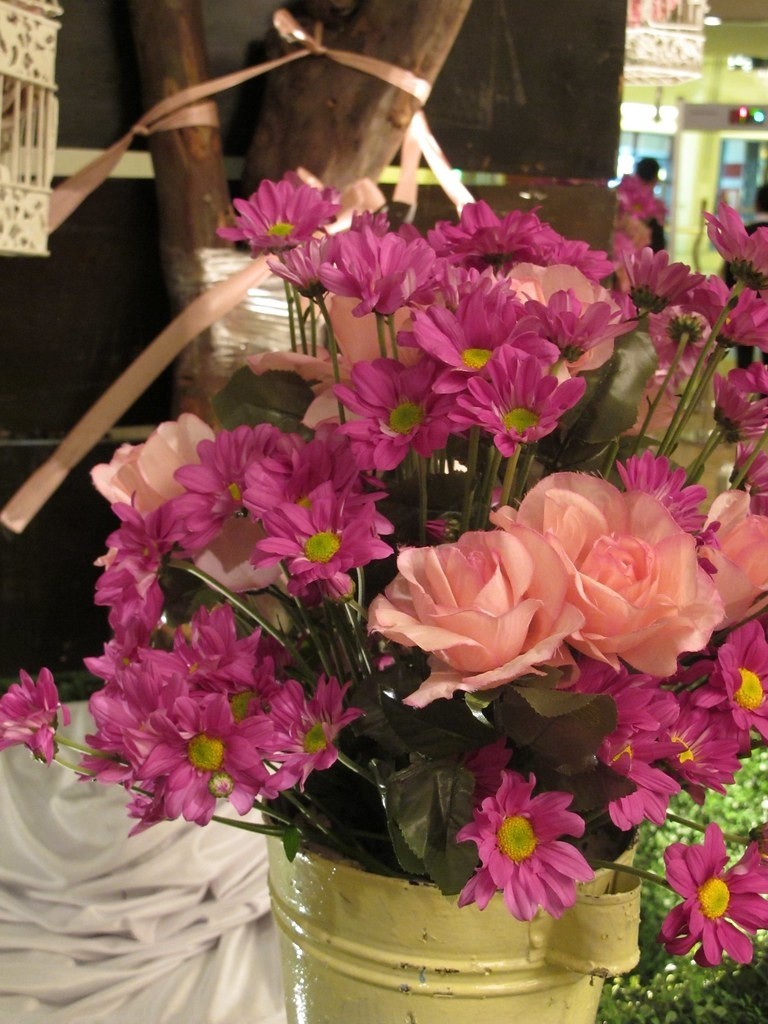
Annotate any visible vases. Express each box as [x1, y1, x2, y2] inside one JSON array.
[[259, 791, 646, 1024]]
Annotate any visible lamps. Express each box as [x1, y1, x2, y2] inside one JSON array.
[[621, 0, 711, 86]]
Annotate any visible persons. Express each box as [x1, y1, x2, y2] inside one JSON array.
[[722, 183, 768, 400], [634, 158, 666, 254]]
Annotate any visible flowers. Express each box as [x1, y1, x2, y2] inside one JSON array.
[[0, 167, 768, 971]]
